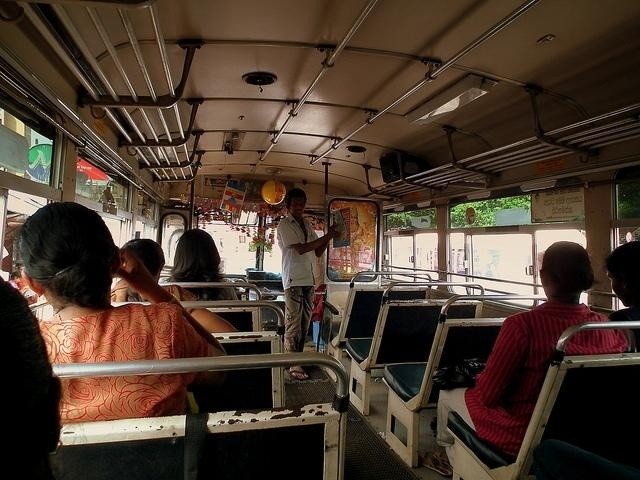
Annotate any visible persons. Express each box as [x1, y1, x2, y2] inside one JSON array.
[[109, 239, 242, 333], [604, 240, 640, 355], [9, 272, 38, 317], [420, 241, 631, 477], [2, 279, 64, 479], [15, 200, 230, 420], [279, 186, 344, 382], [166, 229, 241, 300]]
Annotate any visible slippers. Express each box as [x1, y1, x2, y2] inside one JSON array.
[[289, 366, 309, 380], [417, 448, 454, 478]]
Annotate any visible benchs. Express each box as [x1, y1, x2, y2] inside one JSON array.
[[434, 320, 639, 477], [49, 352, 351, 479], [382, 292, 550, 468], [115, 300, 290, 413], [111, 272, 485, 397]]
